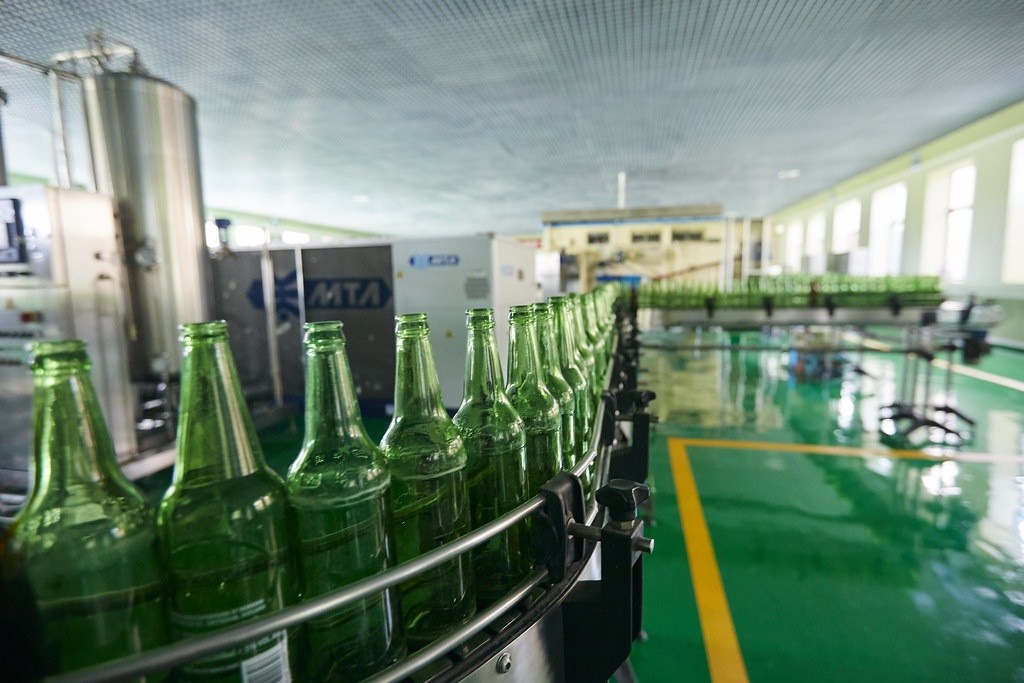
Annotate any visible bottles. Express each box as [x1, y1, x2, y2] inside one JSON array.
[[2, 273, 948, 682]]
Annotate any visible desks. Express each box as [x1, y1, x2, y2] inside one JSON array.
[[877, 326, 984, 442]]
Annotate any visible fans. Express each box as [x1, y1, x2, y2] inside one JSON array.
[[935, 299, 1004, 426]]
